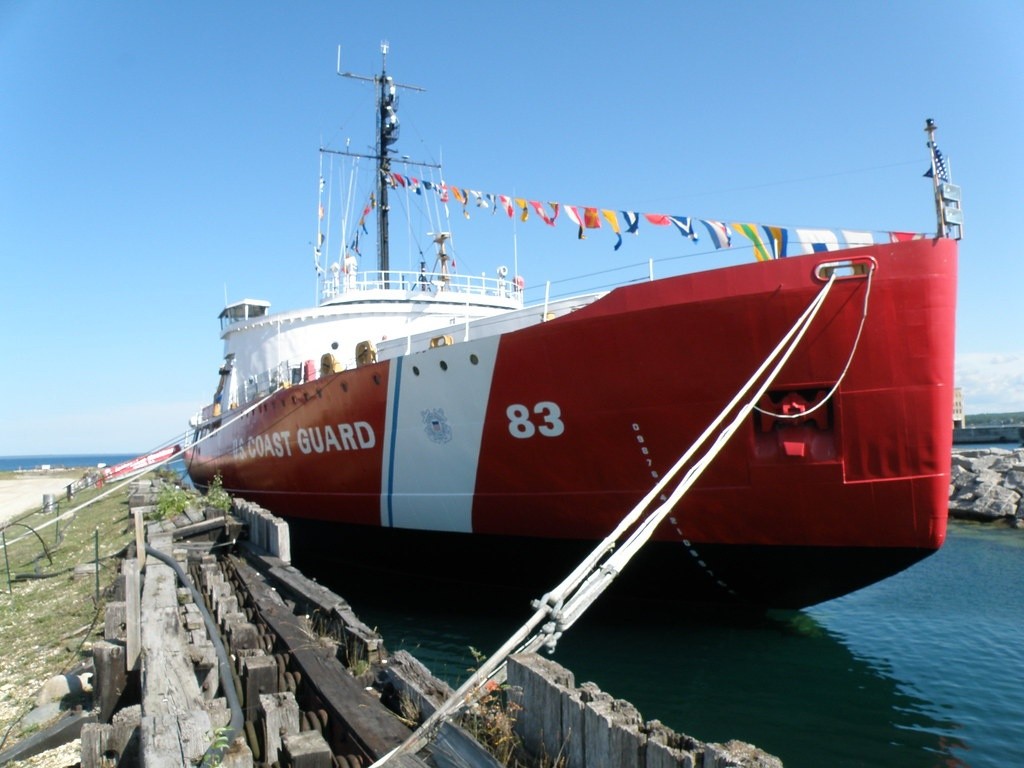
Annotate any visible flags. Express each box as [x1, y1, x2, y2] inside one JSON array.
[[922, 142, 950, 184], [381, 169, 927, 262]]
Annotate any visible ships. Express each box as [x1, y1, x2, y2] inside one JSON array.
[[186, 40, 961, 630]]
